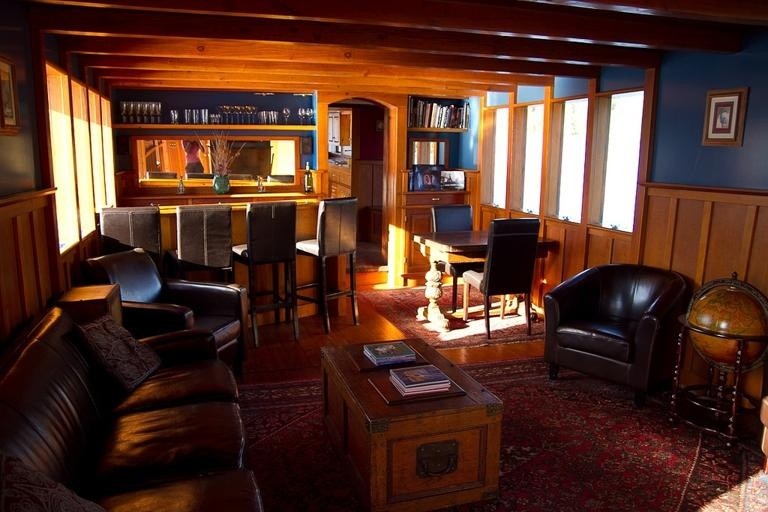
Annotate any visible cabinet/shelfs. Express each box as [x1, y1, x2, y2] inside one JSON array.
[[404, 194, 472, 274], [405, 94, 473, 194], [327, 103, 372, 207], [59, 283, 123, 328]]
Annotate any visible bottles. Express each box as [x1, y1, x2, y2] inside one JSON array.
[[304, 161, 313, 193]]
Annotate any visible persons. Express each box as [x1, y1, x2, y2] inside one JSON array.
[[719, 107, 730, 130], [447, 173, 454, 182], [181, 139, 205, 179], [425, 176, 430, 184]]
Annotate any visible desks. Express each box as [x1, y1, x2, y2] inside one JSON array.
[[408, 229, 560, 332]]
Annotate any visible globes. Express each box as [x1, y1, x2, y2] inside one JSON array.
[[667, 271, 768, 447]]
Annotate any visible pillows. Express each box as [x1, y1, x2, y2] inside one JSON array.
[[0, 455, 126, 512], [72, 313, 164, 397]]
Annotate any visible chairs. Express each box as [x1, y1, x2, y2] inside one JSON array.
[[431, 202, 541, 339], [541, 264, 691, 406], [233, 195, 361, 347], [99, 204, 235, 275], [83, 246, 251, 375]]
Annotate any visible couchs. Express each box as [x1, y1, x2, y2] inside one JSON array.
[[0, 303, 264, 512]]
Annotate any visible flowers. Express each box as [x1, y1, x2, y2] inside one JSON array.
[[189, 128, 249, 176]]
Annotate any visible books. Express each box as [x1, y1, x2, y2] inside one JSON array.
[[365, 341, 451, 397], [408, 96, 467, 165]]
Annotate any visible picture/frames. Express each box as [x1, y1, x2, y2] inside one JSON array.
[[0, 56, 24, 138], [700, 86, 750, 150]]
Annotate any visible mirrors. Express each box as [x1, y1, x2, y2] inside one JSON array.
[[408, 136, 451, 172], [130, 134, 304, 189]]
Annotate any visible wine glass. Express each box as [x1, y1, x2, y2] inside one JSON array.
[[283, 107, 313, 125], [219, 105, 257, 124]]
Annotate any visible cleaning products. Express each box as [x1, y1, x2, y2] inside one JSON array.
[[304, 160, 312, 192]]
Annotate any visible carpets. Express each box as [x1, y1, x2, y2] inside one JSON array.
[[358, 283, 546, 348], [232, 356, 767, 512]]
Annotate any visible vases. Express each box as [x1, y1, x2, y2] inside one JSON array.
[[212, 174, 230, 193]]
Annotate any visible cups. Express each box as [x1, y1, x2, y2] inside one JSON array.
[[170, 108, 209, 124]]
